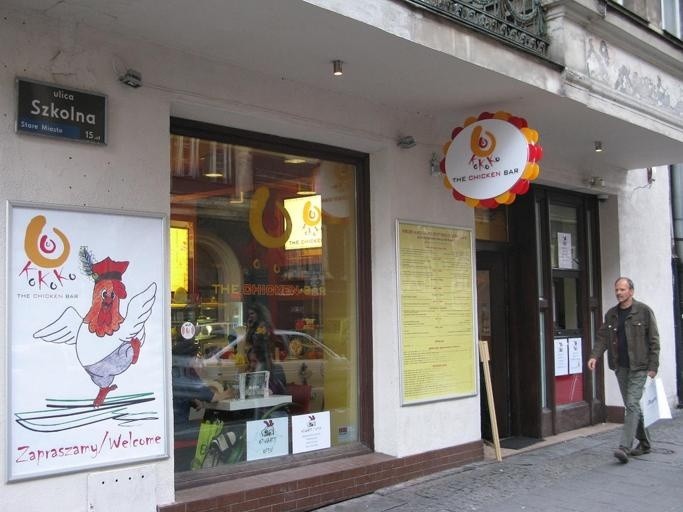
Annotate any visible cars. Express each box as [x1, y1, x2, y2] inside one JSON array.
[[171, 327, 352, 420]]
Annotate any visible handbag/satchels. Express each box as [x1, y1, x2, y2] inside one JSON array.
[[286, 382, 310, 413], [194, 424, 236, 470], [643, 376, 672, 430]]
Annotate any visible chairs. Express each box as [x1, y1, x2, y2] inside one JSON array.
[[174, 383, 314, 452]]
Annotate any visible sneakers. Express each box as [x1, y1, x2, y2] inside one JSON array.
[[631, 443, 651, 455], [615, 444, 630, 465]]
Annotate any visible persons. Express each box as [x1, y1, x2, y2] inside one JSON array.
[[171, 341, 230, 442], [585, 277, 660, 464], [243, 345, 286, 398], [245, 305, 276, 391]]
